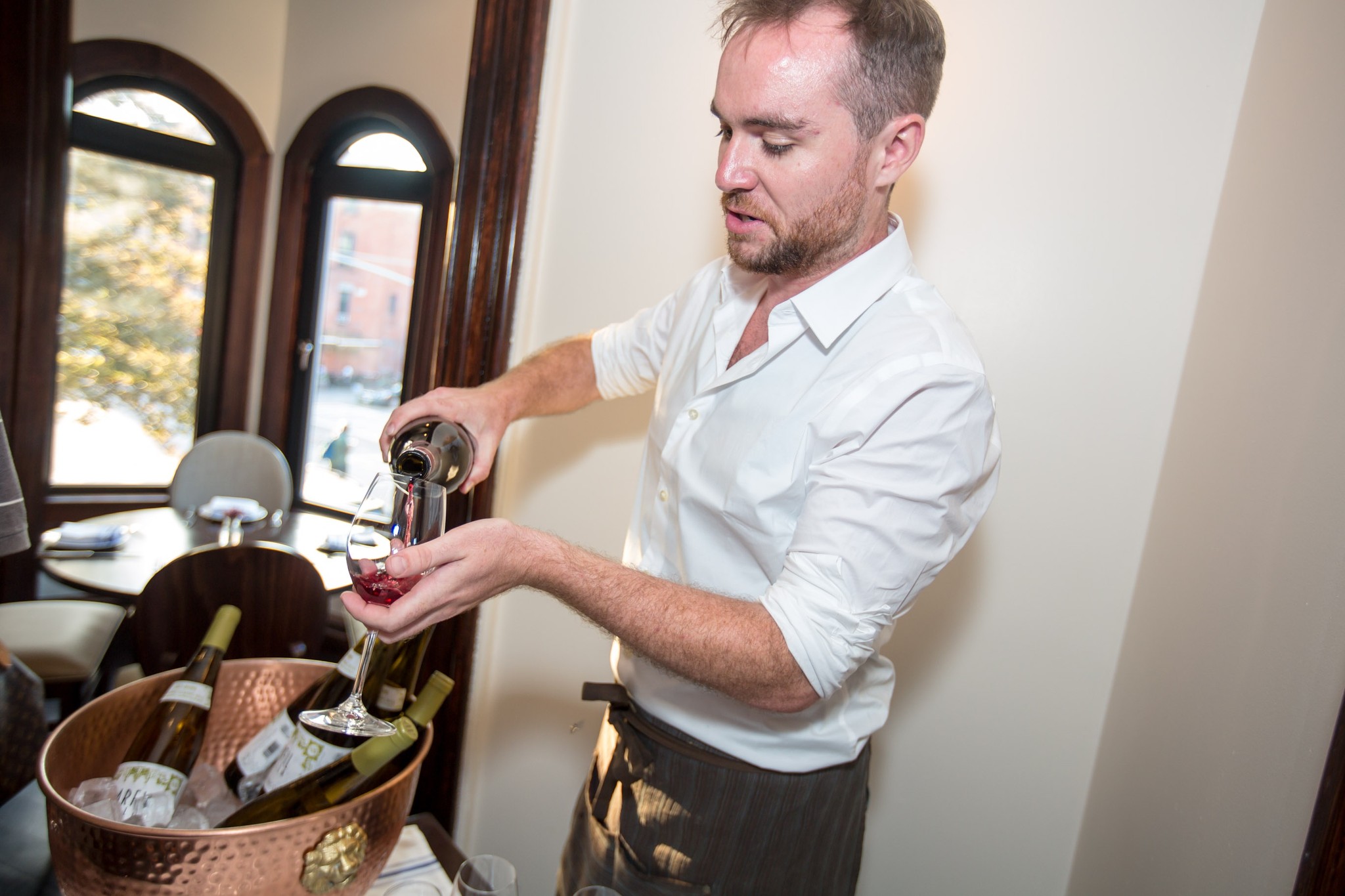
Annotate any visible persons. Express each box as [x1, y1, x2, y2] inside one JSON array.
[[338, 0, 999, 896]]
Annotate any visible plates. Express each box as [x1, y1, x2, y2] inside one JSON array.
[[41, 528, 129, 551]]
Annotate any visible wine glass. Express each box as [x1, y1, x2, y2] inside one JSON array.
[[298, 472, 448, 738]]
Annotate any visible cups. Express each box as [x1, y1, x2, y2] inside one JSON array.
[[451, 855, 517, 896], [384, 880, 442, 896], [574, 885, 622, 896]]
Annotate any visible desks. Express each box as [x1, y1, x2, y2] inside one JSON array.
[[35, 502, 391, 610]]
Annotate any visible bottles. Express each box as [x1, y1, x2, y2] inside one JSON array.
[[111, 603, 243, 828], [388, 417, 474, 498], [254, 621, 439, 805], [215, 717, 418, 827], [222, 673, 331, 797], [220, 512, 245, 545], [338, 669, 454, 807], [198, 503, 268, 523]]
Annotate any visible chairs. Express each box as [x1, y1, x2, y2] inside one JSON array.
[[167, 429, 294, 509], [84, 540, 325, 702]]
